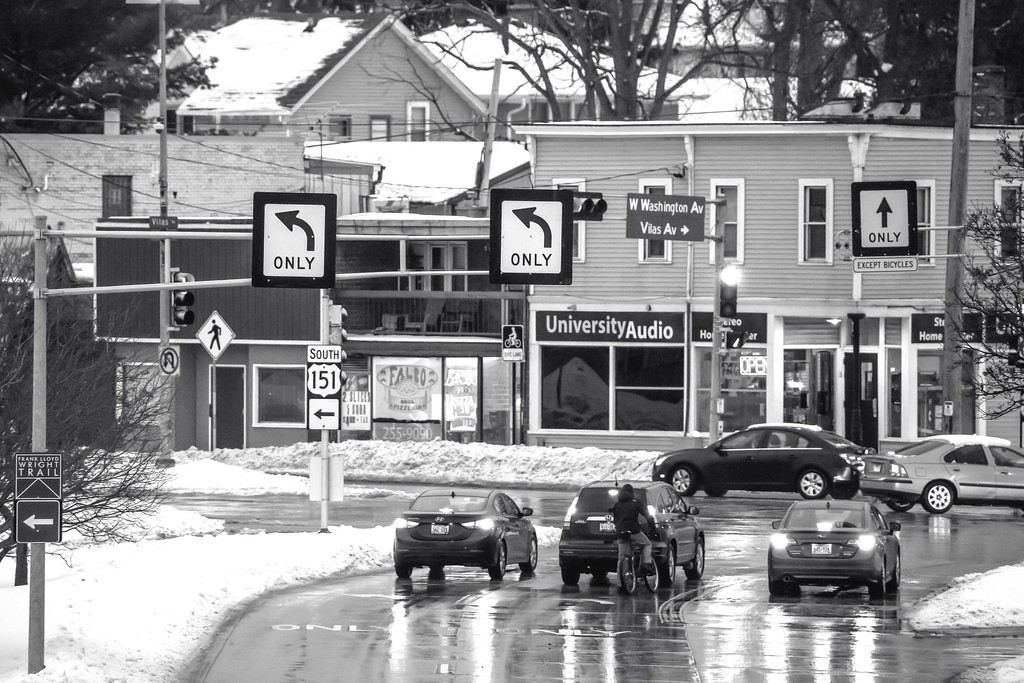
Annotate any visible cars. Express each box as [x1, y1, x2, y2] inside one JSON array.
[[858, 436, 1024, 513], [767, 502, 900, 599], [393, 489, 538, 579], [651, 424, 876, 501]]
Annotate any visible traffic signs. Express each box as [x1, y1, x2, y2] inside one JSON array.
[[850, 182, 918, 257], [253, 192, 337, 290], [490, 189, 571, 285], [628, 193, 705, 243]]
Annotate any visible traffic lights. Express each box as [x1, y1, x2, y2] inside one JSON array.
[[169, 271, 196, 327], [717, 268, 738, 320], [330, 305, 348, 386], [573, 190, 606, 224]]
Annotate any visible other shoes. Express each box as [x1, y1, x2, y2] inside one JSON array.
[[618, 587, 625, 594], [640, 565, 655, 575]]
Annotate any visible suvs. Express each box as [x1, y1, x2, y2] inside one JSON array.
[[559, 480, 705, 588]]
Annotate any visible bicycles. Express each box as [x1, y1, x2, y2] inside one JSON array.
[[619, 530, 660, 595]]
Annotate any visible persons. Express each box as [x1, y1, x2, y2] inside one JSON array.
[[613, 484, 656, 594]]
